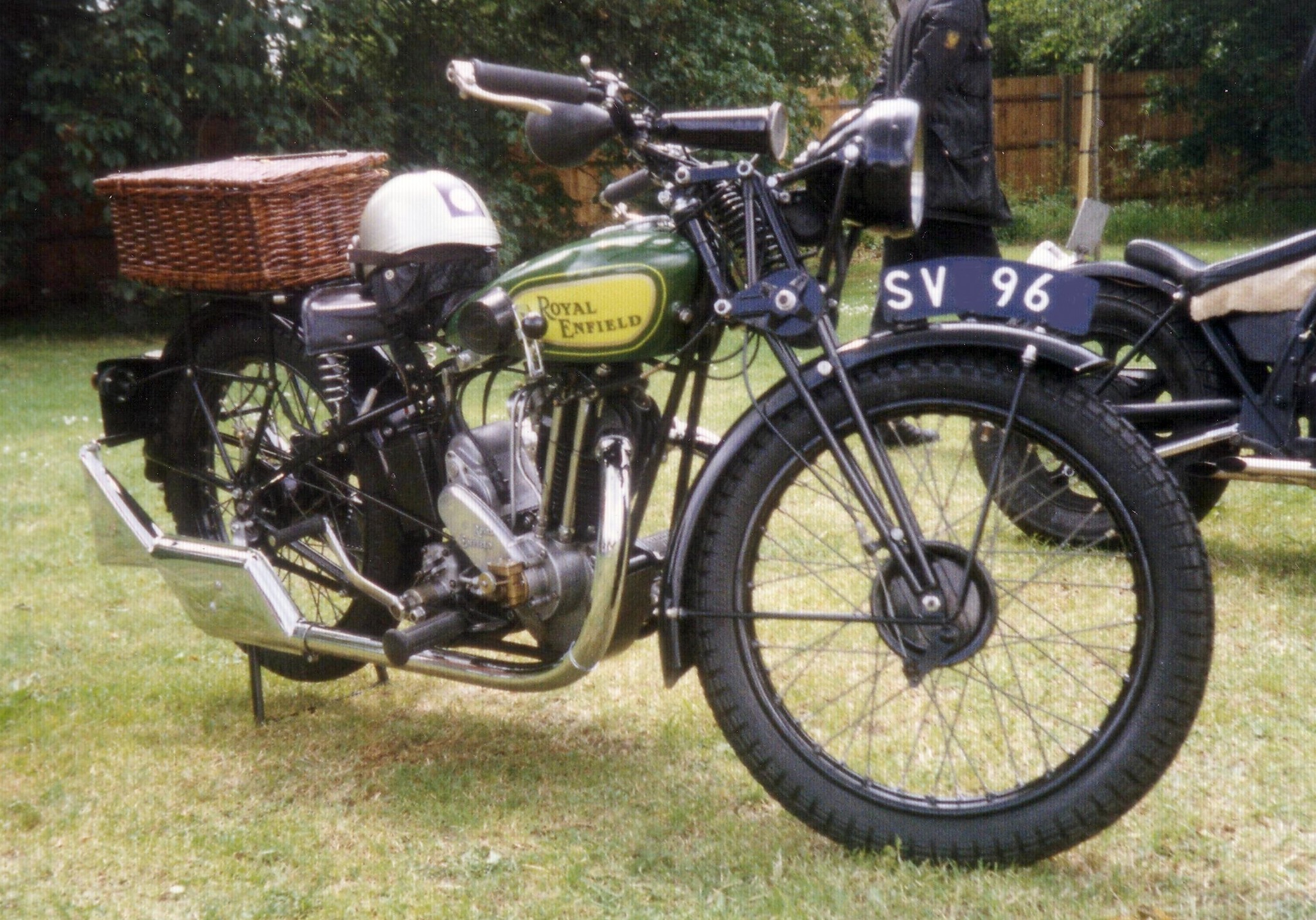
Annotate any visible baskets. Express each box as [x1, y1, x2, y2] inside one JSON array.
[[92, 150, 390, 293]]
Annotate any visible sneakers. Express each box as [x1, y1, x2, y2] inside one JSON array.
[[869, 419, 940, 449]]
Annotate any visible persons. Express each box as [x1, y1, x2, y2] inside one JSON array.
[[868, 0, 1014, 445]]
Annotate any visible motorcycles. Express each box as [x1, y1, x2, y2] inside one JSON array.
[[75, 52, 1213, 871], [965, 228, 1316, 540]]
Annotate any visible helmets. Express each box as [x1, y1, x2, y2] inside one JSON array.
[[348, 171, 502, 326]]
